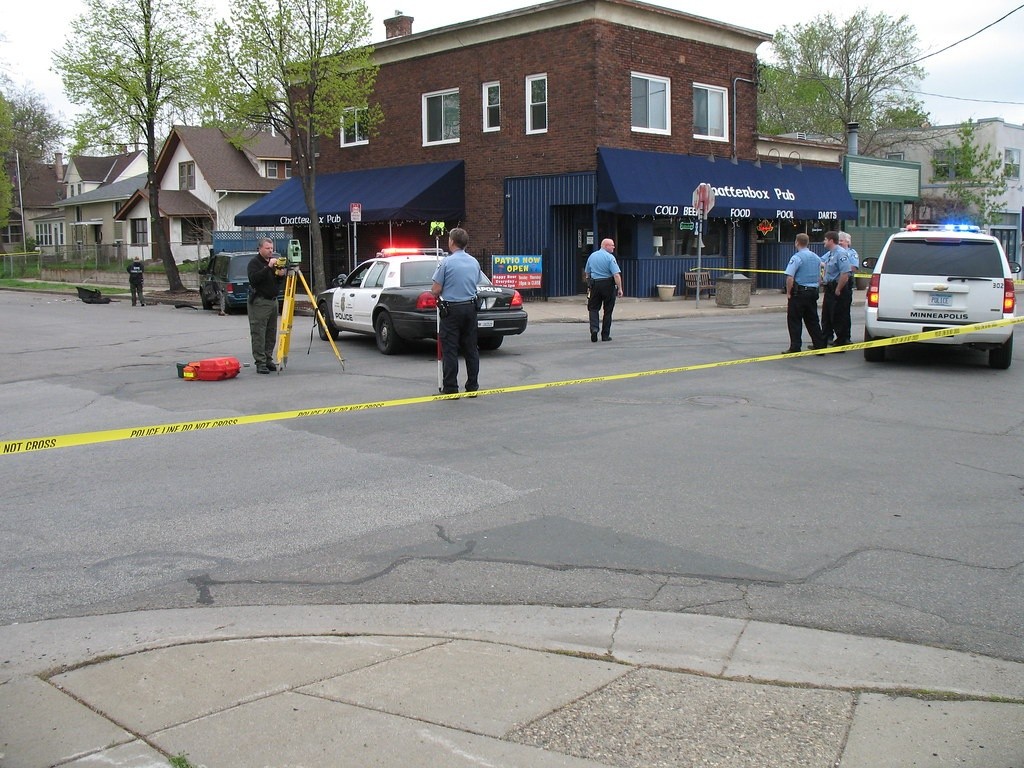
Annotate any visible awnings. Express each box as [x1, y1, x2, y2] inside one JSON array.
[[596, 145, 859, 309], [234, 157, 468, 311]]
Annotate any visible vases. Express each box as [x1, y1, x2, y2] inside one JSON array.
[[657, 284, 676, 301], [855, 278, 868, 289]]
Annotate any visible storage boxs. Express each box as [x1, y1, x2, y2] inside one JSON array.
[[197, 356, 241, 381]]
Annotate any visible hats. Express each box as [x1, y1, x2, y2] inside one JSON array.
[[134, 256, 140, 262]]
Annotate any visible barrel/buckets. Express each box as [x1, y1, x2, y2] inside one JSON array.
[[657, 285, 676, 301]]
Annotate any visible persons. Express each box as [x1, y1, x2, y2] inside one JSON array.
[[585, 238, 623, 342], [247, 238, 285, 374], [431, 228, 482, 400], [127, 257, 146, 306], [781, 231, 859, 358]]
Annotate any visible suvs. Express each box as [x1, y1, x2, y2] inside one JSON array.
[[860, 223, 1021, 370], [199, 251, 287, 316]]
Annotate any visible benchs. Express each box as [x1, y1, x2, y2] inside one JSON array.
[[685, 272, 717, 300]]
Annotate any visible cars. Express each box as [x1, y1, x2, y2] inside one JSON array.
[[312, 247, 527, 355]]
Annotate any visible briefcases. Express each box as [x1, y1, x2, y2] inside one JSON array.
[[199, 358, 241, 381]]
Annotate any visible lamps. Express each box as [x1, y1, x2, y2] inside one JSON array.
[[688, 141, 803, 173], [653, 236, 663, 257]]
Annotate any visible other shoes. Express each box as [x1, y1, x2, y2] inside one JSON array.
[[257, 366, 270, 374], [782, 349, 801, 354], [828, 336, 834, 345], [602, 335, 612, 341], [132, 303, 136, 306], [807, 344, 816, 350], [848, 340, 854, 344], [465, 390, 477, 398], [591, 329, 598, 342], [267, 361, 282, 371], [432, 387, 459, 400], [141, 303, 144, 306]]
